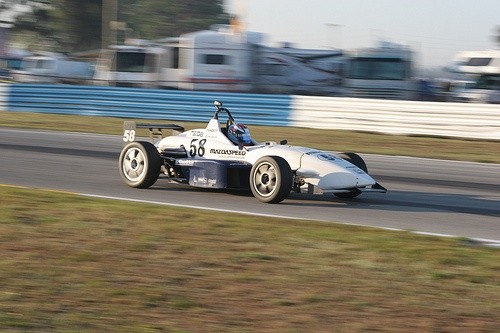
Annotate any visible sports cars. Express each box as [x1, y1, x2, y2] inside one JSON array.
[[119, 101, 390, 205]]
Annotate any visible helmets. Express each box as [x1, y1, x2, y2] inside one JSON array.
[[227, 123, 251, 146]]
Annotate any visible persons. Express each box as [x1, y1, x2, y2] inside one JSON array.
[[227, 122, 252, 148]]
